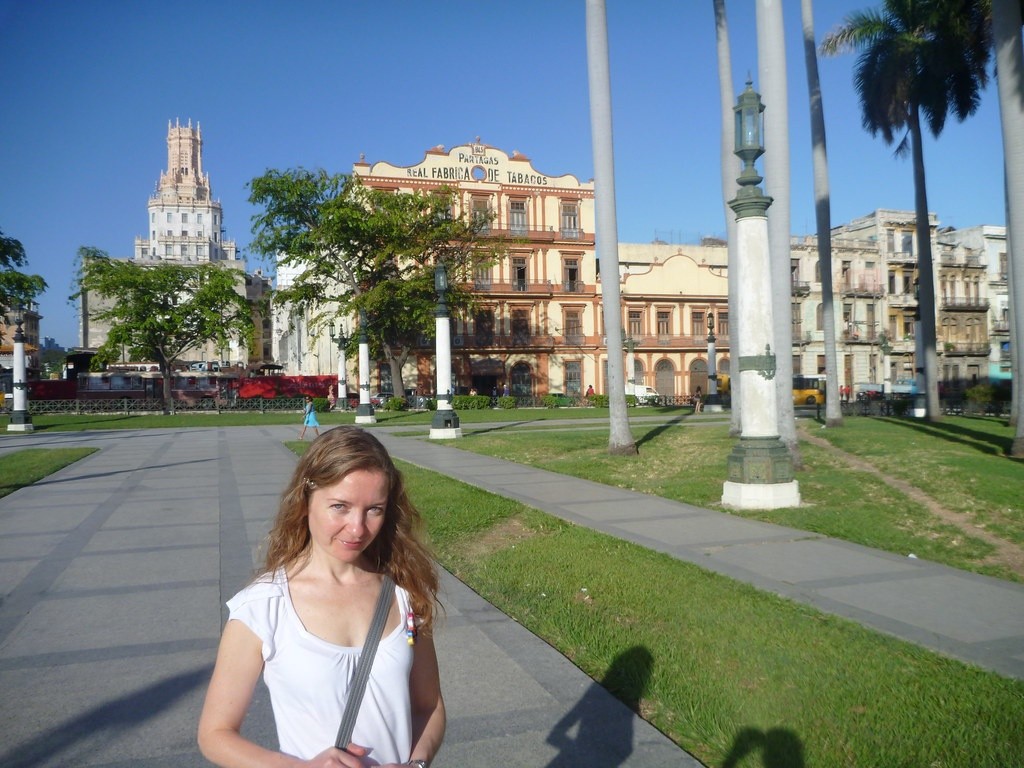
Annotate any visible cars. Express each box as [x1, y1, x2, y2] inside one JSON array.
[[371, 391, 395, 407], [348, 393, 379, 411], [405, 386, 428, 409]]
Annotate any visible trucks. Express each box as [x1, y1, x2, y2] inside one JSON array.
[[625, 382, 662, 408]]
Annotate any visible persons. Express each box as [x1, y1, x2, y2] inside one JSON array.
[[503, 385, 509, 397], [938, 381, 947, 415], [492, 387, 497, 397], [840, 385, 850, 407], [198, 424, 446, 768], [586, 385, 594, 406], [469, 387, 477, 396], [297, 395, 320, 440], [693, 386, 703, 413]]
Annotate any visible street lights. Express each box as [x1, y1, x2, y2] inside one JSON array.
[[720, 70, 804, 515], [7, 308, 35, 433], [333, 322, 352, 412], [429, 254, 464, 442], [355, 307, 378, 425]]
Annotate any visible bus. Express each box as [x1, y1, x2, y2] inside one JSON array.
[[713, 371, 828, 406]]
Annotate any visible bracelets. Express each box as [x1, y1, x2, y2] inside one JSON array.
[[407, 759, 427, 768]]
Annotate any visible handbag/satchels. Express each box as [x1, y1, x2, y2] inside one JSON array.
[[328, 396, 334, 401]]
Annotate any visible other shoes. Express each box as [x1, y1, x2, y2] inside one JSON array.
[[296, 437, 302, 440]]
